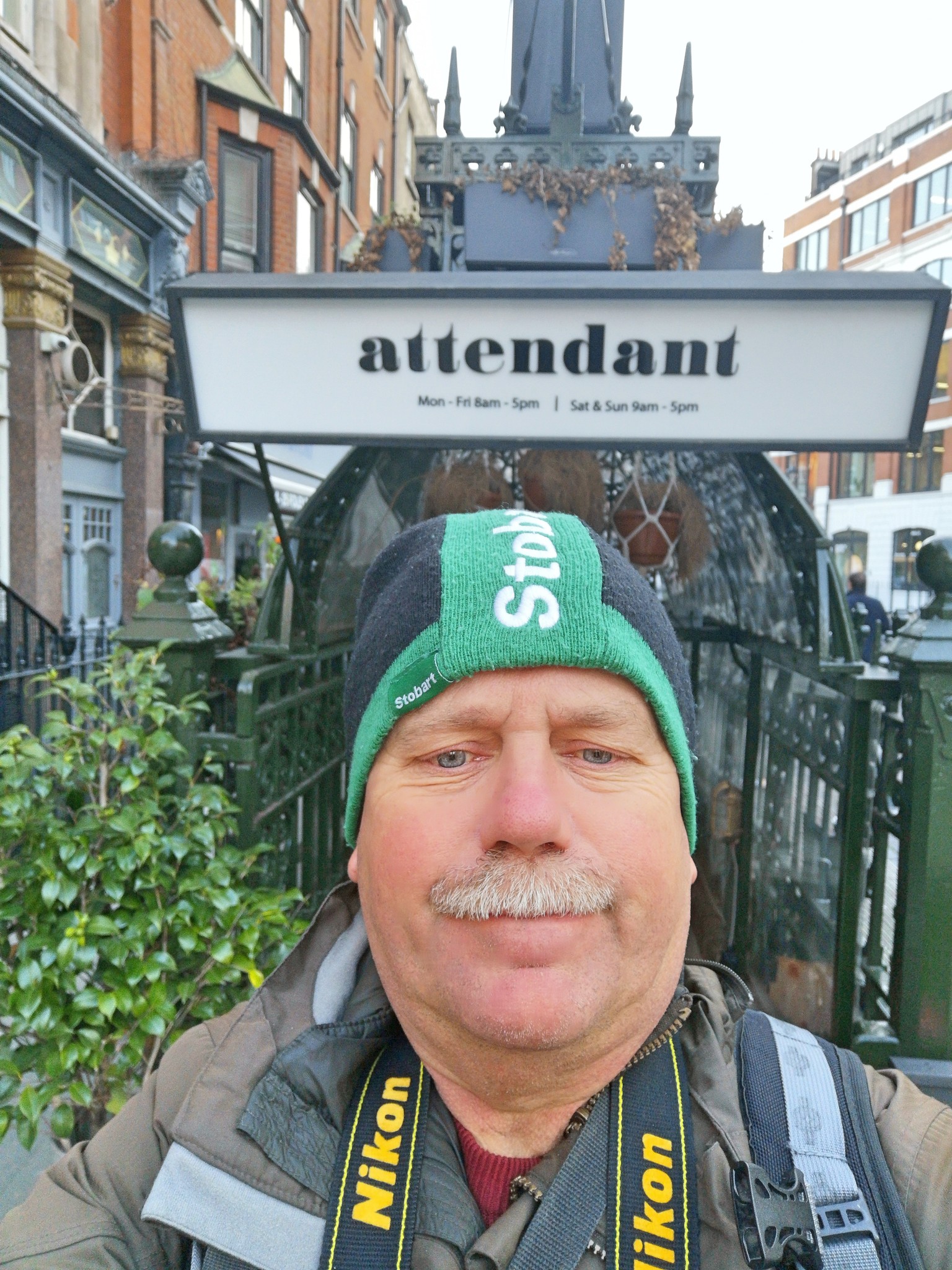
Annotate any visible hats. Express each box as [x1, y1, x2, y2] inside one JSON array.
[[345, 510, 698, 857]]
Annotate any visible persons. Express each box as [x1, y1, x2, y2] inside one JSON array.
[[0, 505, 952, 1269], [841, 572, 893, 667]]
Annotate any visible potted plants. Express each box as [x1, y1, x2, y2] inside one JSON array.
[[416, 464, 513, 523], [695, 205, 766, 271], [610, 482, 713, 581], [350, 217, 430, 272], [518, 448, 606, 533], [462, 159, 701, 272]]
